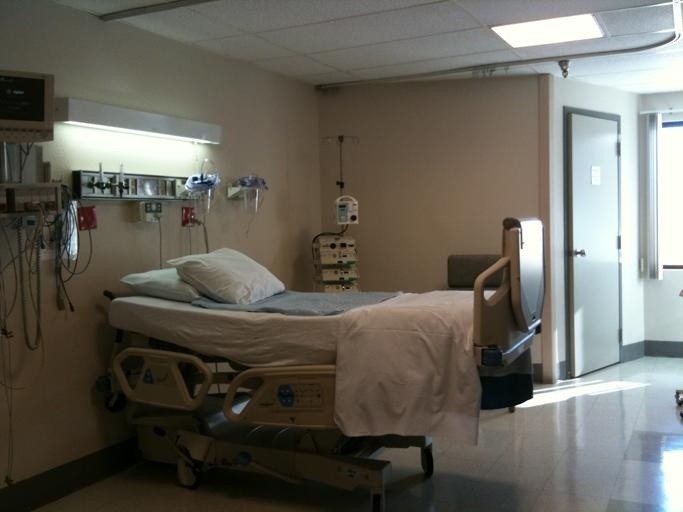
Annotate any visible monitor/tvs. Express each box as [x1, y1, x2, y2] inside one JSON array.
[[0, 69, 54, 143]]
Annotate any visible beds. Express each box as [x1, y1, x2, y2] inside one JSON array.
[[100, 215, 546, 512]]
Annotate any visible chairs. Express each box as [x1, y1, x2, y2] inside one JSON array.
[[445, 251, 506, 292]]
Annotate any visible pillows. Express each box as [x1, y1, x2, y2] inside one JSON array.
[[118, 248, 287, 307]]
